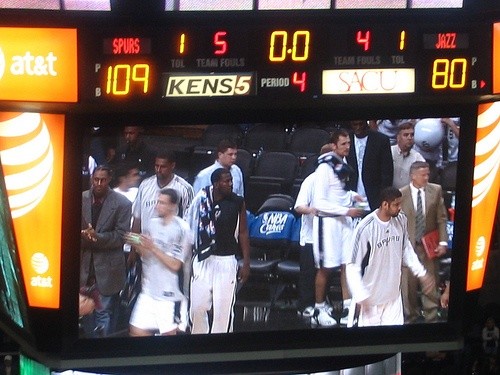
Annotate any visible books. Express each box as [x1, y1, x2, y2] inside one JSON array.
[[422, 230, 438, 259]]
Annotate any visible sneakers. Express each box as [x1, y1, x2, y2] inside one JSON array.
[[310, 309, 337, 326], [340, 306, 350, 324]]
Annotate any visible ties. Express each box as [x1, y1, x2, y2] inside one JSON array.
[[415, 191, 425, 243]]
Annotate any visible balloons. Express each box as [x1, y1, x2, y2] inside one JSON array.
[[413, 118, 445, 149]]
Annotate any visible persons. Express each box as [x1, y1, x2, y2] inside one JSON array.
[[369, 118, 460, 220], [295, 142, 338, 316], [122, 188, 194, 337], [348, 185, 435, 326], [105, 126, 157, 190], [397, 161, 448, 325], [113, 163, 141, 310], [193, 139, 244, 243], [343, 119, 394, 279], [310, 129, 365, 327], [183, 167, 251, 334], [125, 150, 194, 336], [78, 164, 133, 340]]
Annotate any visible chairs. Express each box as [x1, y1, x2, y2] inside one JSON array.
[[440, 161, 457, 191], [79, 118, 331, 309]]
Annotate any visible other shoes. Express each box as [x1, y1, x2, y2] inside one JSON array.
[[297, 306, 315, 316]]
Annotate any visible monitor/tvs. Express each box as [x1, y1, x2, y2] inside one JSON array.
[[56, 106, 477, 357]]
